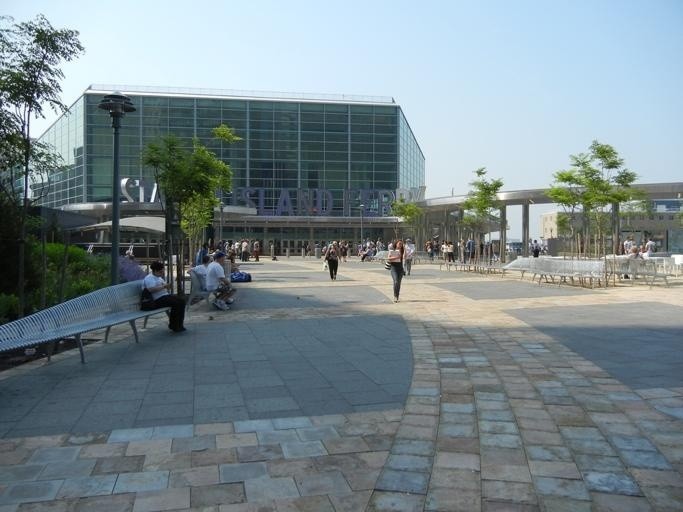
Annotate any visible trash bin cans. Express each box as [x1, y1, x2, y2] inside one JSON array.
[[221, 260, 231, 284], [315, 248, 321, 259]]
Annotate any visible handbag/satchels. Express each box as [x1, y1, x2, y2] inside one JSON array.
[[140, 288, 155, 311], [323, 261, 329, 272], [384, 261, 392, 270]]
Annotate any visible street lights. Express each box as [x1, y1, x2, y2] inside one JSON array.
[[103, 88, 126, 283]]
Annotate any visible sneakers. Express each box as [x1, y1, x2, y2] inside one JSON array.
[[168, 324, 186, 332], [211, 297, 234, 311]]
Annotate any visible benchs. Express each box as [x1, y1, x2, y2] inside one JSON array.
[[372, 251, 433, 264], [186, 264, 212, 315], [502, 253, 682, 289], [439, 261, 502, 274], [0, 279, 172, 365]]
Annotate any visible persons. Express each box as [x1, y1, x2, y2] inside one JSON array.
[[425, 238, 492, 263], [623, 235, 656, 279], [531, 240, 541, 257], [403, 239, 416, 275], [321, 237, 393, 262], [141, 261, 188, 333], [323, 241, 342, 280], [388, 240, 405, 303], [303, 242, 313, 260], [125, 250, 135, 260], [187, 238, 259, 312]]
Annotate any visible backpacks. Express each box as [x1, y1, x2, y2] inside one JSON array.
[[230, 271, 251, 282]]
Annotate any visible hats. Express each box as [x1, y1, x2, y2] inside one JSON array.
[[213, 251, 228, 259]]
